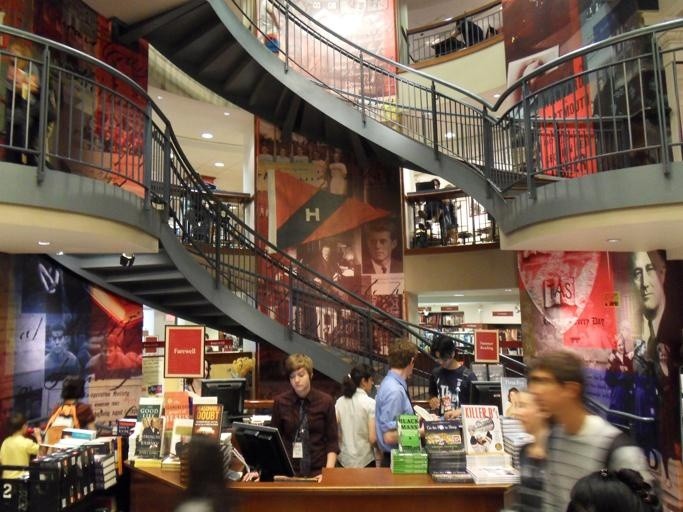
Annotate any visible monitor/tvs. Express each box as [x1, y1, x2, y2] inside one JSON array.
[[201, 375, 246, 422], [468, 379, 502, 418], [236, 424, 296, 479]]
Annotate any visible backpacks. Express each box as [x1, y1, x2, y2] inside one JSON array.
[[37, 404, 81, 453]]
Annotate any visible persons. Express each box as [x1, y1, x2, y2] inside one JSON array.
[[4, 39, 57, 168], [375, 339, 425, 468], [526, 350, 660, 512], [259, 0, 281, 58], [428, 335, 478, 420], [567, 467, 664, 512], [604, 333, 679, 464], [239, 352, 340, 483], [335, 363, 384, 469], [313, 245, 340, 344], [629, 250, 683, 358], [362, 221, 403, 274], [44, 320, 141, 379], [418, 179, 445, 226], [514, 389, 553, 512], [50, 375, 95, 430], [451, 19, 484, 46], [260, 144, 348, 197]]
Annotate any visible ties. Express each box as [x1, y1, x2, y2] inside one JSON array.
[[298, 399, 312, 475], [381, 267, 387, 273], [647, 319, 654, 337]]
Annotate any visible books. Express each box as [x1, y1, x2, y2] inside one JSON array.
[[391, 376, 536, 486], [0, 392, 234, 512]]
